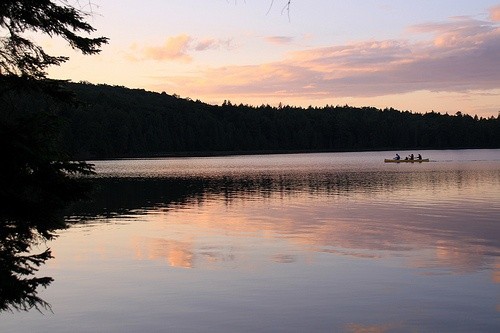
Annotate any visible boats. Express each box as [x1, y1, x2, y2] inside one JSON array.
[[384, 158, 429, 163]]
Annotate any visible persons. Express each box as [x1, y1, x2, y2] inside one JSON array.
[[394, 154, 400, 160], [408, 154, 413, 160], [416, 154, 422, 161], [405, 157, 408, 160]]
